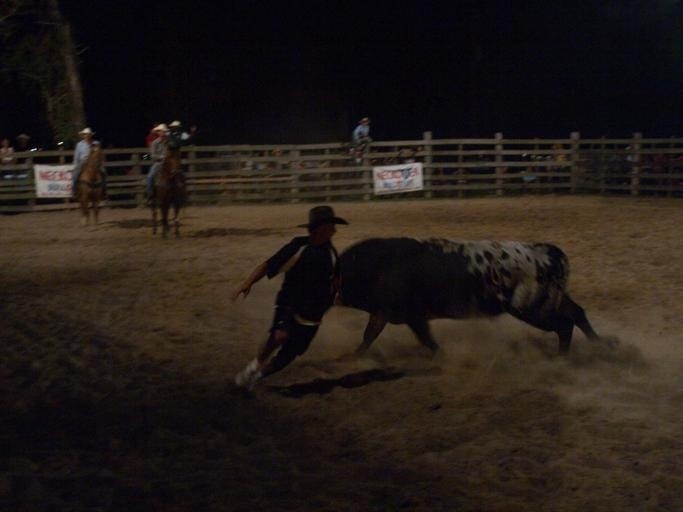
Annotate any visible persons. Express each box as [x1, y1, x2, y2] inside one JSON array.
[[350, 117, 371, 155], [0, 139, 14, 162], [230, 205, 349, 393], [146, 123, 162, 145], [142, 123, 185, 208], [14, 134, 30, 164], [67, 126, 109, 200], [168, 120, 197, 142]]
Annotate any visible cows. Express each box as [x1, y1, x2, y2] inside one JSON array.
[[332, 233, 602, 361]]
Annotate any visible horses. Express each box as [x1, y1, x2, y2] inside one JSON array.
[[75, 139, 106, 227], [146, 134, 186, 240]]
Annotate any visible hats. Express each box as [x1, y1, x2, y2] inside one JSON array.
[[15, 134, 29, 140], [150, 123, 169, 132], [294, 205, 348, 228], [167, 120, 181, 128], [77, 127, 95, 137], [358, 117, 371, 123]]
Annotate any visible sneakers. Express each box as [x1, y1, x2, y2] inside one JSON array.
[[233, 365, 256, 391]]
